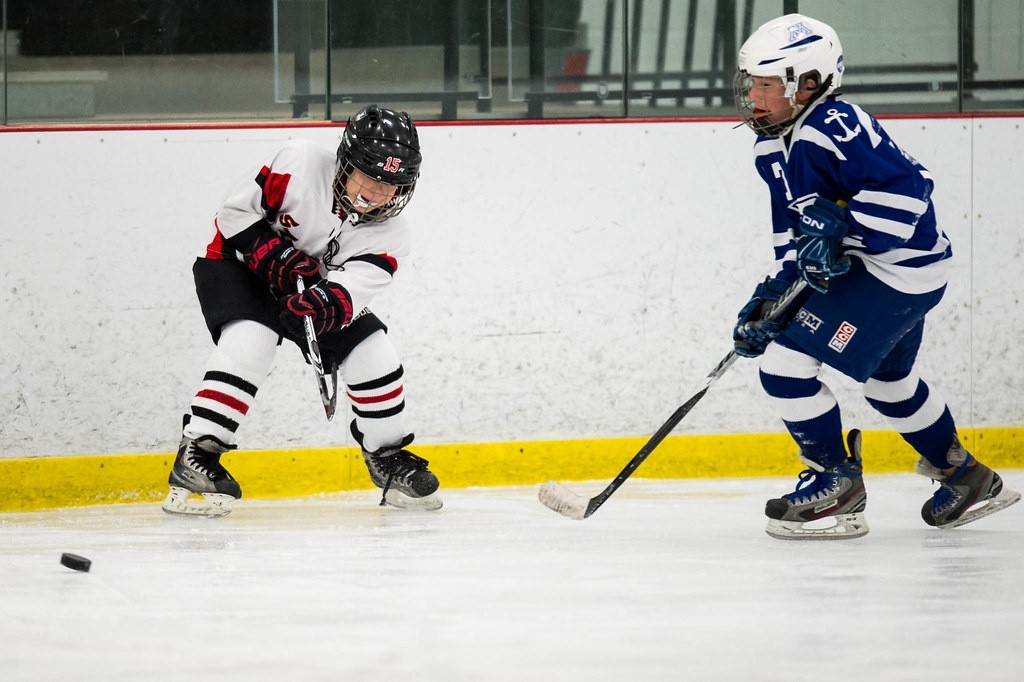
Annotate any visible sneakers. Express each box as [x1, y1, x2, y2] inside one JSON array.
[[350, 418, 443, 510], [917, 432, 1022, 529], [163, 413, 242, 516], [765, 429, 870, 538]]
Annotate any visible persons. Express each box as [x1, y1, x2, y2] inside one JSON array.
[[160, 104, 451, 516], [733, 15, 1022, 540]]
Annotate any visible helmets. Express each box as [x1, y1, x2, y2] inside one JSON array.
[[738, 13, 846, 135], [334, 104, 421, 222]]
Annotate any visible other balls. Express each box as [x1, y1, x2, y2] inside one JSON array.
[[60, 553, 92, 573]]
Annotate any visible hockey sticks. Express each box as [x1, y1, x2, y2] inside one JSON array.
[[296, 274, 338, 421], [537, 276, 809, 523]]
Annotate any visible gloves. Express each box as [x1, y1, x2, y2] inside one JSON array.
[[280, 279, 352, 339], [243, 230, 320, 303], [794, 205, 851, 293], [733, 275, 795, 357]]
